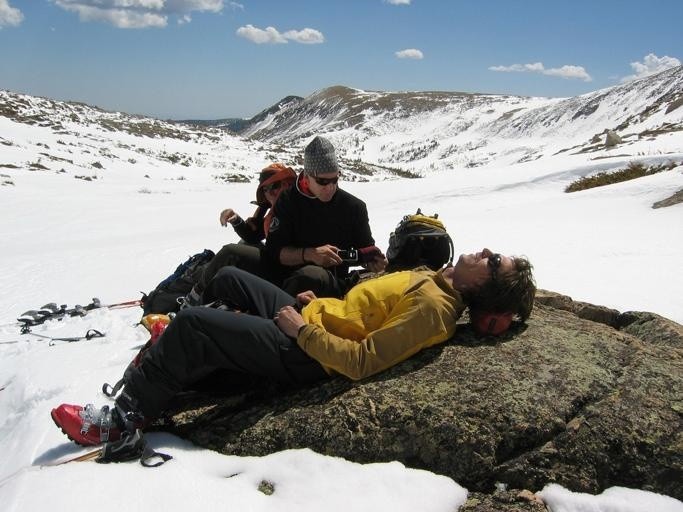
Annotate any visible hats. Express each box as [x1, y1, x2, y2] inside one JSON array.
[[304, 136, 339, 176]]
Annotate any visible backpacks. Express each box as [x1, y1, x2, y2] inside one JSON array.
[[386, 208, 454, 273], [143, 249, 216, 317]]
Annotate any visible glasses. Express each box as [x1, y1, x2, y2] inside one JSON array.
[[312, 175, 339, 186], [487, 254, 501, 280], [262, 181, 281, 192]]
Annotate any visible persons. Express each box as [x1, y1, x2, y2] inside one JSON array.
[[216, 161, 296, 245], [141, 135, 390, 335], [51, 247, 538, 450]]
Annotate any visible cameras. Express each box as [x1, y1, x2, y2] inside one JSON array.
[[336, 247, 359, 262]]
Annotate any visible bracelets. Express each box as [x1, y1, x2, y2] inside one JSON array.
[[295, 324, 307, 336], [301, 245, 308, 263]]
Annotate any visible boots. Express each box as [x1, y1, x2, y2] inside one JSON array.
[[51, 403, 146, 446], [141, 314, 172, 344]]
